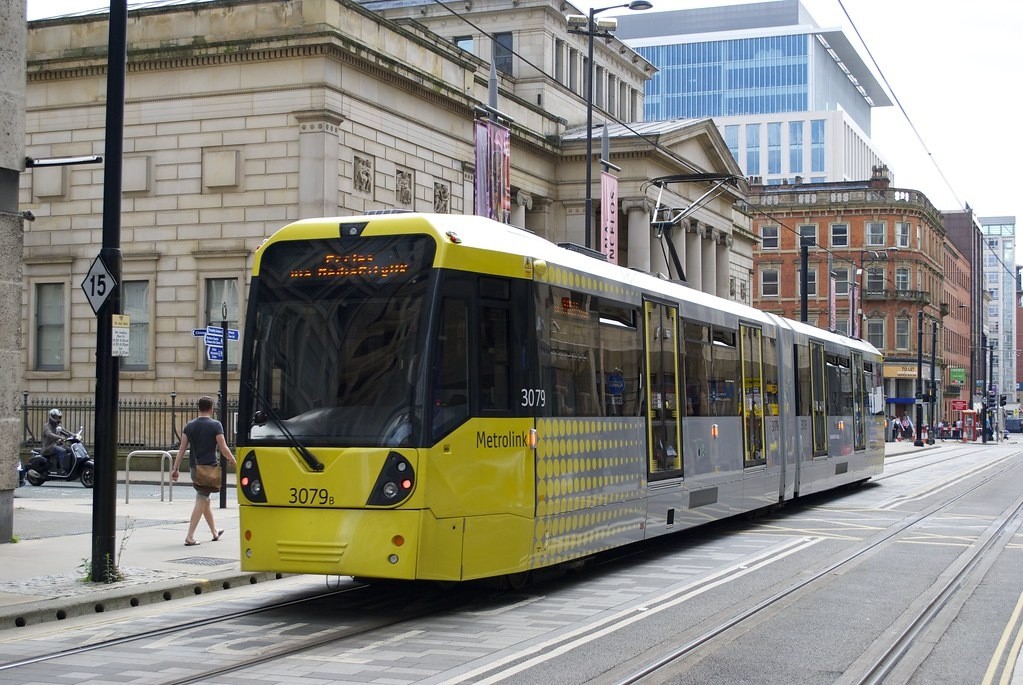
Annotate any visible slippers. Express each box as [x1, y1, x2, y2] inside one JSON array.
[[212, 531, 224, 541], [185, 540, 201, 546]]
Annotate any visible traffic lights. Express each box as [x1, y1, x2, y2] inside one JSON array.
[[1000, 395, 1007, 406], [988, 394, 995, 408]]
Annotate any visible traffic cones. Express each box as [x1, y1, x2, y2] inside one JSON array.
[[897, 431, 901, 442], [962, 428, 967, 443], [911, 427, 928, 443]]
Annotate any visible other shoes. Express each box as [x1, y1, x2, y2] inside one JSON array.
[[57, 468, 66, 475]]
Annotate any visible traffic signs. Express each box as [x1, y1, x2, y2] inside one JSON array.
[[192, 325, 240, 361]]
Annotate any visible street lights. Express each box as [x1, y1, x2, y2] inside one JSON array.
[[859, 246, 900, 341], [580, 0, 654, 249]]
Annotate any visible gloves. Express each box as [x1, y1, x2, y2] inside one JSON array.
[[59, 437, 64, 442]]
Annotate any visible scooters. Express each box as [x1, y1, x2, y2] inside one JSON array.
[[25, 425, 95, 488]]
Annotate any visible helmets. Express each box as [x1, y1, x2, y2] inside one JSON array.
[[48, 409, 62, 427]]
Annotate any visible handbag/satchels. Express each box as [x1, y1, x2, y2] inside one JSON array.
[[191, 464, 222, 490], [894, 424, 899, 429]]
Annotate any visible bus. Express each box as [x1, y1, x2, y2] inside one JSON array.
[[231, 170, 889, 591]]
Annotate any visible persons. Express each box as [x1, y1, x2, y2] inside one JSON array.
[[933, 414, 993, 442], [42, 408, 73, 475], [171, 396, 238, 546], [885, 410, 913, 442]]
